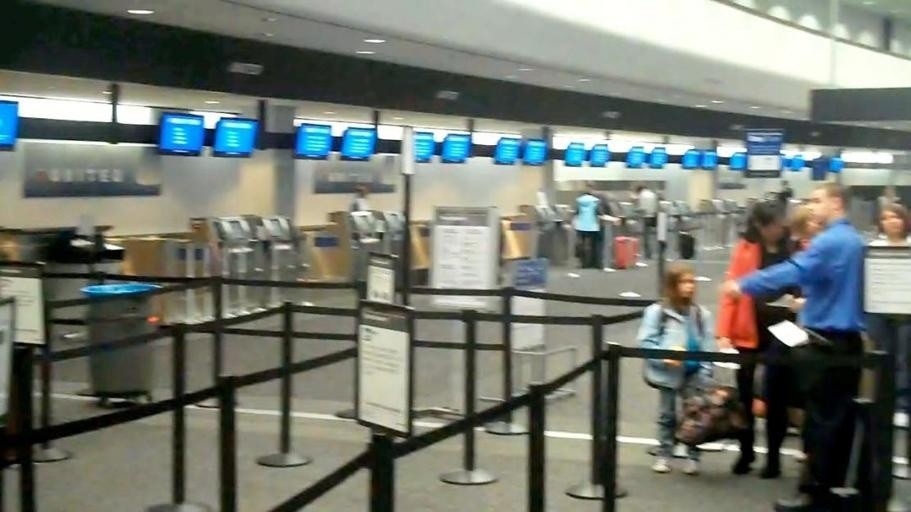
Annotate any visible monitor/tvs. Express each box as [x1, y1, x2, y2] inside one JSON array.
[[493, 137, 522, 166], [157, 112, 205, 157], [565, 143, 842, 172], [0, 100, 19, 152], [340, 126, 377, 161], [440, 133, 472, 163], [414, 131, 435, 163], [213, 118, 260, 158], [293, 123, 332, 159], [522, 138, 548, 165]]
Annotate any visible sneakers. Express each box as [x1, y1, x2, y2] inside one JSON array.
[[652, 455, 781, 478]]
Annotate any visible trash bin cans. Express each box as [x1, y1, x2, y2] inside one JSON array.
[[80, 284, 166, 406], [615, 236, 641, 269], [678, 230, 696, 260]]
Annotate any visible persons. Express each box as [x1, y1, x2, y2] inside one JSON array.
[[351, 185, 370, 210], [574, 180, 613, 269], [635, 184, 659, 260], [636, 261, 715, 476]]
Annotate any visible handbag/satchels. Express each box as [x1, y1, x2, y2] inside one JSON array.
[[674, 386, 747, 444]]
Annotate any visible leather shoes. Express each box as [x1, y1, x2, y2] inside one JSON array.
[[775, 495, 813, 511]]
